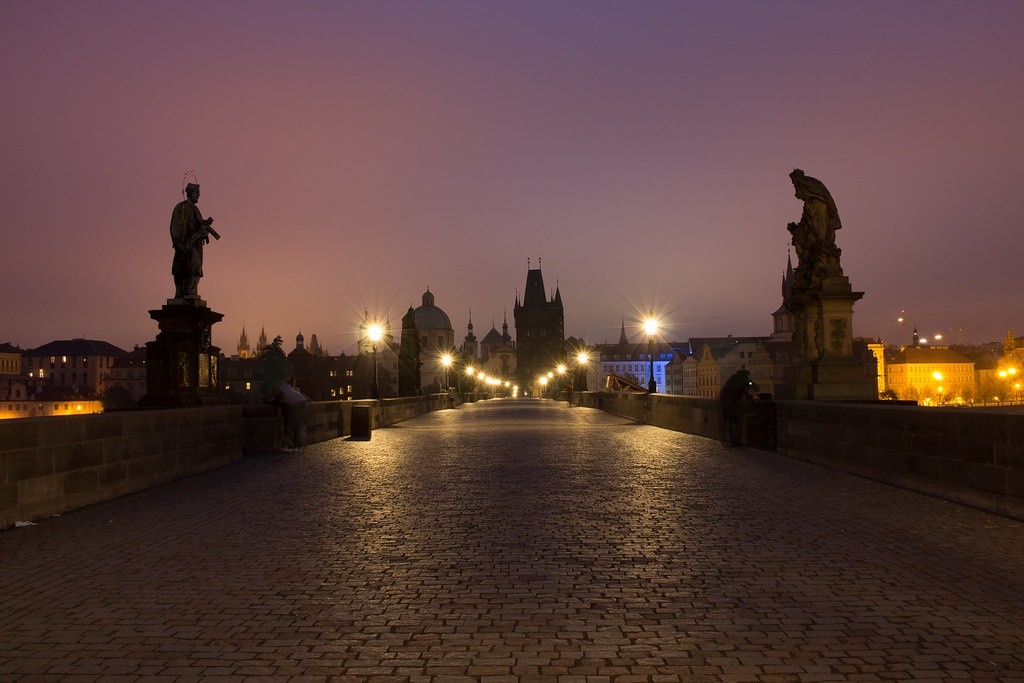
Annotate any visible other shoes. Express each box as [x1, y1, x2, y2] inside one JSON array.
[[281, 445, 299, 452], [726, 441, 745, 448]]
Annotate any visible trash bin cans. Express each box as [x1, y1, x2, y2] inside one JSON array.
[[350, 405, 372, 441]]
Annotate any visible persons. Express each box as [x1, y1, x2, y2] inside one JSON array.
[[270, 375, 309, 452], [170, 183, 221, 298], [721, 369, 758, 450], [786, 169, 842, 268]]
